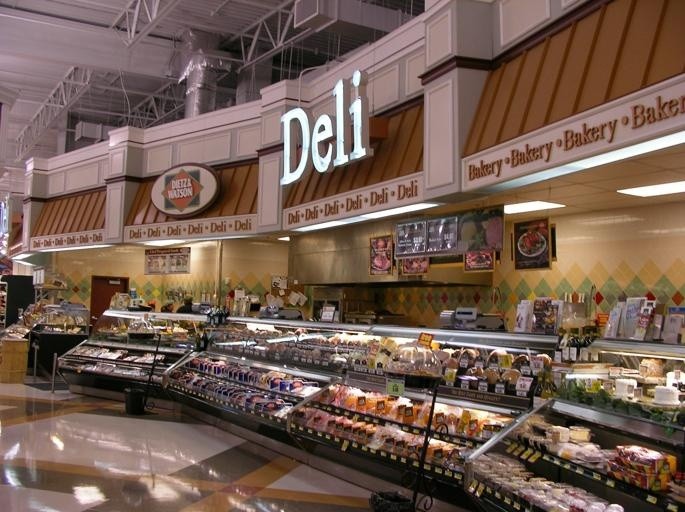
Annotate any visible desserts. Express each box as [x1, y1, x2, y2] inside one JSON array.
[[0, 304, 685, 512]]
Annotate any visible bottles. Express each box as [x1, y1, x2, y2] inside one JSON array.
[[225, 295, 251, 317], [555, 331, 599, 362]]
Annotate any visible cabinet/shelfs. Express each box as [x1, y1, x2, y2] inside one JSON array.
[[57, 309, 212, 415], [287, 315, 560, 511], [1, 300, 92, 383], [463, 317, 684, 512], [162, 317, 371, 458]]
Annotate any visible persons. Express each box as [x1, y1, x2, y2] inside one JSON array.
[[175, 295, 194, 313], [161, 299, 175, 313]]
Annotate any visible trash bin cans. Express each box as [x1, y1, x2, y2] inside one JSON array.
[[125, 389, 145, 414], [370, 492, 414, 512]]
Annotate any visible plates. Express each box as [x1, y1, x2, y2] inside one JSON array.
[[517, 232, 547, 257], [649, 399, 682, 406], [466, 253, 493, 268]]
